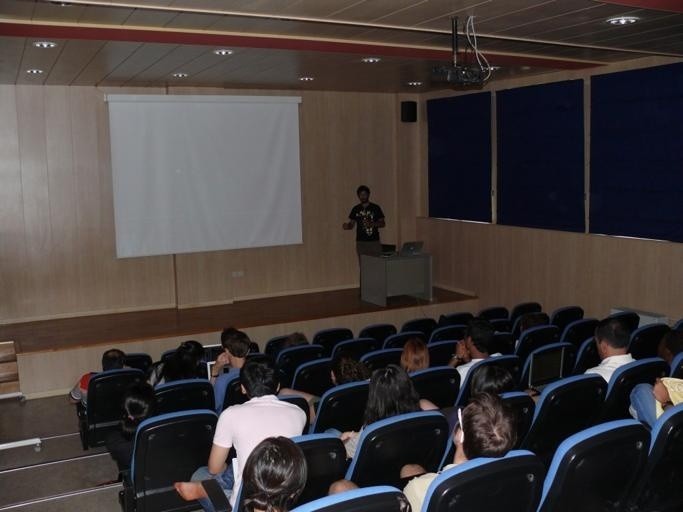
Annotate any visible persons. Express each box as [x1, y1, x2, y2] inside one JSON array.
[[342, 186, 385, 297], [657, 330, 683, 361], [325, 364, 424, 475], [221, 327, 238, 347], [328, 391, 518, 512], [242, 435, 307, 512], [629, 378, 675, 431], [158, 351, 199, 385], [68, 349, 132, 416], [472, 360, 519, 400], [147, 340, 204, 387], [583, 318, 637, 384], [447, 320, 504, 390], [400, 338, 430, 374], [173, 357, 307, 512], [277, 332, 308, 348], [279, 355, 369, 435], [209, 331, 251, 416]]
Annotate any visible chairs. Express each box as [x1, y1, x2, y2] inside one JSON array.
[[603, 358, 669, 421], [288, 357, 335, 394], [602, 310, 638, 334], [203, 344, 224, 361], [345, 409, 450, 486], [399, 318, 437, 333], [359, 323, 397, 338], [608, 17, 637, 28], [277, 394, 308, 435], [382, 331, 429, 349], [149, 377, 215, 416], [313, 327, 353, 346], [522, 341, 575, 390], [625, 323, 672, 360], [248, 342, 259, 354], [427, 340, 460, 368], [670, 351, 683, 380], [561, 317, 598, 342], [524, 371, 606, 451], [331, 338, 380, 363], [454, 355, 524, 409], [573, 336, 603, 375], [673, 318, 683, 332], [291, 483, 412, 512], [74, 369, 145, 450], [272, 342, 324, 375], [511, 312, 550, 333], [533, 419, 651, 512], [124, 353, 152, 370], [438, 313, 474, 328], [420, 446, 540, 511], [381, 244, 397, 252], [549, 305, 584, 326], [265, 332, 310, 353], [308, 380, 374, 435], [161, 349, 176, 359], [511, 301, 541, 318], [218, 373, 251, 413], [359, 347, 403, 377], [405, 365, 464, 411], [230, 434, 345, 511], [475, 306, 511, 322], [429, 325, 468, 343], [625, 400, 683, 512], [514, 324, 560, 357], [439, 391, 535, 460], [121, 408, 221, 512], [488, 318, 513, 333]]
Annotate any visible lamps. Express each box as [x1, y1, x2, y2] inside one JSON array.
[[406, 80, 421, 87], [173, 72, 189, 79], [362, 57, 379, 64], [33, 41, 56, 50], [300, 76, 316, 83], [26, 68, 45, 74], [213, 47, 234, 57], [487, 65, 502, 73]]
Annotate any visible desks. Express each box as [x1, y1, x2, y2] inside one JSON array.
[[360, 251, 433, 307]]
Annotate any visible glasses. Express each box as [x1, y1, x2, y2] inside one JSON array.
[[457, 404, 465, 430]]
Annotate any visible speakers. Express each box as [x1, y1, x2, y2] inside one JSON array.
[[401, 101, 417, 122]]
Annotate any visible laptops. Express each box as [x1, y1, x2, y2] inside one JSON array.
[[401, 241, 424, 256]]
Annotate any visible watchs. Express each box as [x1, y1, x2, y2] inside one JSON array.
[[661, 401, 673, 410]]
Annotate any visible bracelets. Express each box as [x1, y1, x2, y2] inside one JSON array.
[[450, 354, 460, 360], [211, 374, 219, 377]]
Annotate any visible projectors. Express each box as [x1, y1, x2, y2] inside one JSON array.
[[428, 61, 490, 91]]
